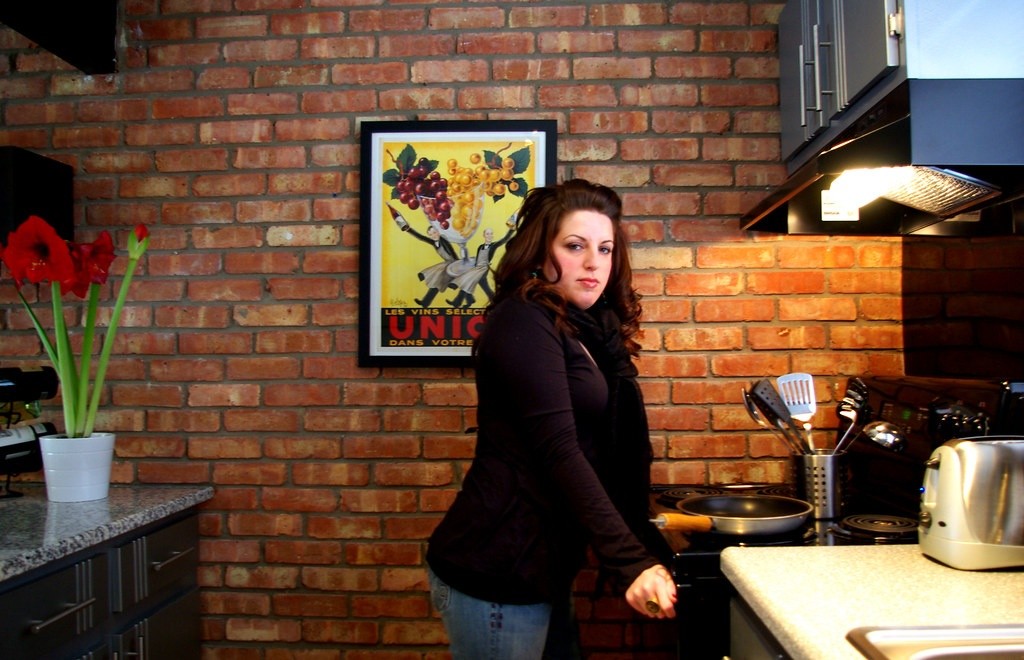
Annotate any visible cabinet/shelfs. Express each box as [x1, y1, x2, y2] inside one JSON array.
[[0, 480, 218, 660], [772, 0, 1024, 177]]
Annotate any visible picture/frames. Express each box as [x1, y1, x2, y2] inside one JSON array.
[[356, 118, 559, 368]]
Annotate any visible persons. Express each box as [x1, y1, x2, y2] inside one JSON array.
[[424, 178, 681, 660]]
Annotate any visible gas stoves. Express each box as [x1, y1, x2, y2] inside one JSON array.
[[638, 483, 922, 580]]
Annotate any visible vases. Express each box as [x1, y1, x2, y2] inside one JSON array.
[[38, 432, 117, 504]]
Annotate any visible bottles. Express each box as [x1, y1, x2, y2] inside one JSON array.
[[0, 366, 59, 401], [0, 422, 58, 475]]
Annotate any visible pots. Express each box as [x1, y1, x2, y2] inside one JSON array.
[[648, 493, 814, 535]]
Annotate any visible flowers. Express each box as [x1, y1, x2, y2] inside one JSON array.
[[0, 215, 152, 438]]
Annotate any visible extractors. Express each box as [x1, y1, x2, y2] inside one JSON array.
[[739, 106, 1024, 237]]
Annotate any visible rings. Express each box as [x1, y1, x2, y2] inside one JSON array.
[[645, 597, 660, 614]]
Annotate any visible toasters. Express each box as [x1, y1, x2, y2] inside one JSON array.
[[917, 434, 1024, 571]]
